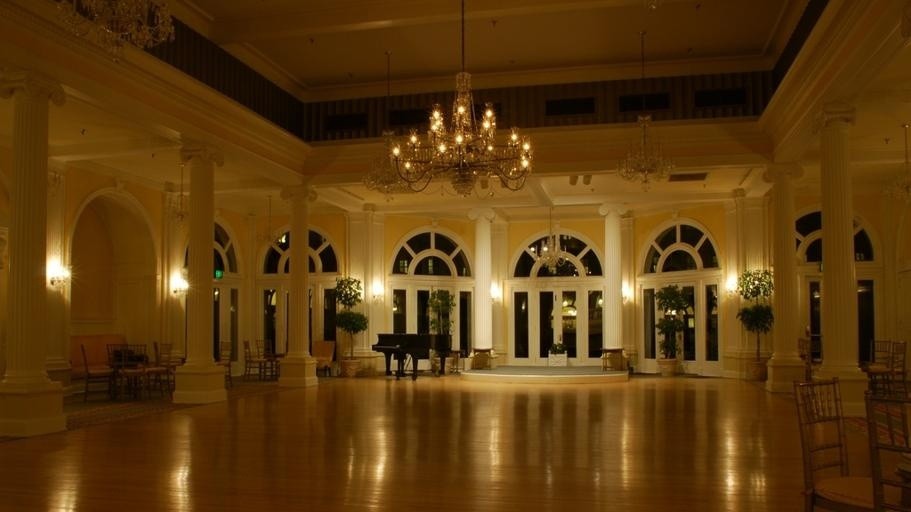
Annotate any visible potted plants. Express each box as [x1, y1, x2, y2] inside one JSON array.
[[426, 290, 456, 374], [333, 277, 367, 377], [653, 285, 690, 376], [549, 343, 567, 366], [736, 269, 776, 380]]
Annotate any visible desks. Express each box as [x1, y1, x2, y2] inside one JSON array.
[[474, 348, 491, 369], [601, 348, 624, 371]]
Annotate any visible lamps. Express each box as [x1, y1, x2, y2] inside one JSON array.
[[539, 207, 567, 267]]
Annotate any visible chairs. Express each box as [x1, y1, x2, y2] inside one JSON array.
[[54, 0, 173, 63], [794, 340, 911, 512], [312, 341, 335, 376], [362, 51, 422, 193], [392, 0, 532, 198], [256, 340, 277, 381], [216, 342, 233, 385], [81, 342, 172, 402], [617, 33, 675, 190], [243, 341, 266, 381]]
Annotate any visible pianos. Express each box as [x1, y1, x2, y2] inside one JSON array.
[[372, 333, 452, 380]]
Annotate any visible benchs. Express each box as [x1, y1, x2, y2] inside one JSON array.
[[435, 350, 465, 373]]
